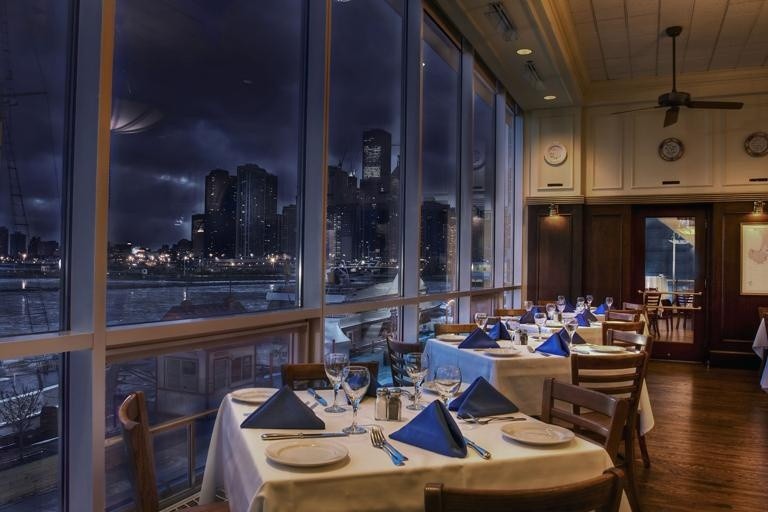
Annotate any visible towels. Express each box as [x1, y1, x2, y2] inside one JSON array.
[[237, 382, 327, 431]]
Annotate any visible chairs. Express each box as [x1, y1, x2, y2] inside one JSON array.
[[753, 307, 767, 391], [413, 468, 629, 512], [117, 390, 230, 512], [566, 349, 649, 512], [643, 288, 701, 339], [276, 292, 654, 469]]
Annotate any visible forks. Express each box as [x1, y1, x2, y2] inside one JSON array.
[[526, 344, 551, 357], [458, 414, 526, 425], [305, 400, 318, 409], [366, 427, 411, 469]]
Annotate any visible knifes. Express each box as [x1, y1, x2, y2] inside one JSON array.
[[572, 349, 589, 355], [260, 431, 350, 440], [322, 350, 351, 413], [460, 433, 492, 461], [307, 386, 328, 406]]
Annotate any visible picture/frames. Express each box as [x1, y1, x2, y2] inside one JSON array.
[[657, 136, 684, 162], [544, 142, 566, 165], [743, 131, 767, 157], [737, 219, 767, 298]]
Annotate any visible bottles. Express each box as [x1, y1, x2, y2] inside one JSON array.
[[374, 386, 402, 421]]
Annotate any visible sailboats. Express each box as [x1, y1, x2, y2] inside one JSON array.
[[0, 0, 120, 469]]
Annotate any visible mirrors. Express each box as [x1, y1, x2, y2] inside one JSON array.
[[640, 212, 704, 349]]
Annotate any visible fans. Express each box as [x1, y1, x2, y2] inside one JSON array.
[[606, 25, 743, 129]]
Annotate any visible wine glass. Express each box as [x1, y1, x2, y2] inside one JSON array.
[[524, 293, 613, 317], [474, 312, 489, 329], [340, 365, 369, 435], [433, 364, 462, 405], [505, 316, 522, 349], [563, 317, 578, 347], [533, 312, 549, 343], [404, 351, 431, 411]]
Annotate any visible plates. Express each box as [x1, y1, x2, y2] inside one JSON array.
[[484, 347, 521, 357], [500, 419, 575, 445], [263, 439, 349, 467], [593, 344, 626, 352], [232, 387, 280, 404], [435, 333, 468, 341], [420, 377, 473, 395]]
